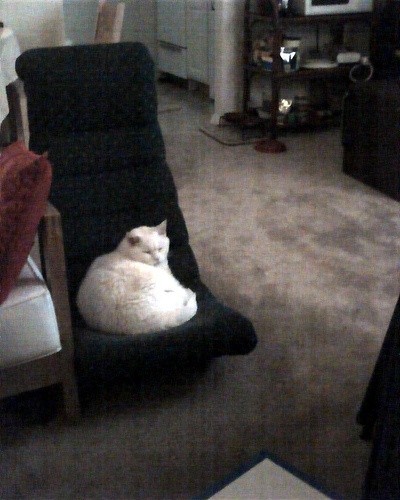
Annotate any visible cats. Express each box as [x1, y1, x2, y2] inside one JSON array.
[[76, 220, 198, 336]]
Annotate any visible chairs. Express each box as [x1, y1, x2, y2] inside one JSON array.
[[0, 84, 81, 426]]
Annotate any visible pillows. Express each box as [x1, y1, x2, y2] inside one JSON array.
[[5, 40, 258, 392], [1, 139, 56, 305]]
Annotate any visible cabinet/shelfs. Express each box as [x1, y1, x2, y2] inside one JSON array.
[[155, 1, 215, 100], [243, 1, 374, 143]]
